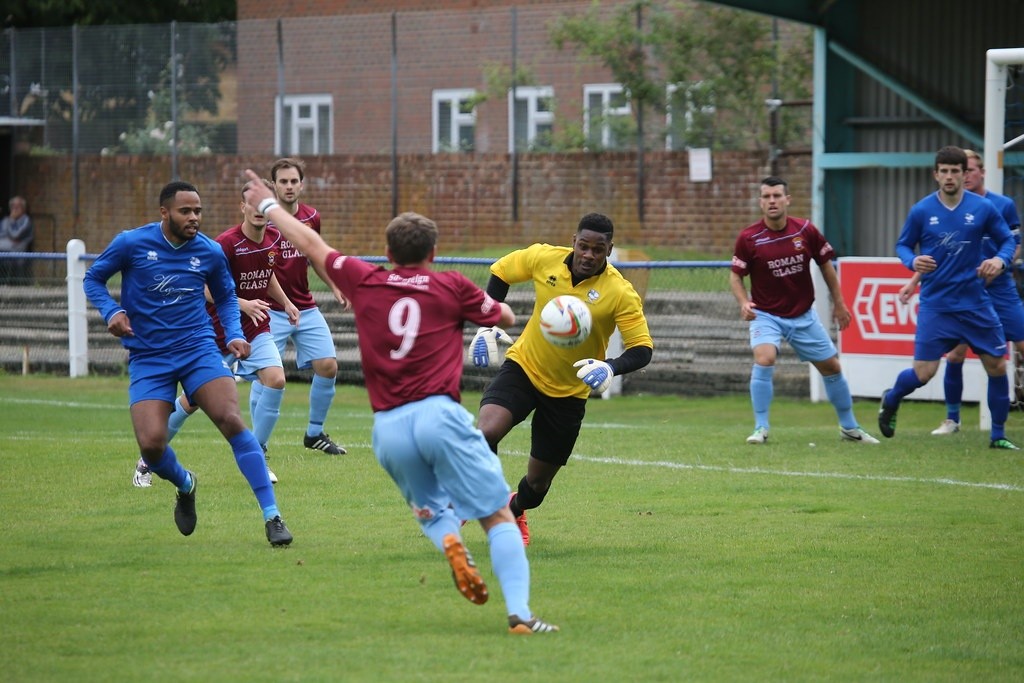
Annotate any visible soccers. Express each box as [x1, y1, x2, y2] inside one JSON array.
[[539, 295, 594, 349]]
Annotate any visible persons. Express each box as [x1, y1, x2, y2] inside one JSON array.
[[896, 149, 1024, 436], [132, 179, 301, 489], [241, 169, 558, 636], [0, 196, 34, 286], [878, 145, 1019, 452], [248, 157, 348, 455], [729, 175, 879, 445], [83, 180, 294, 547], [469, 213, 654, 550]]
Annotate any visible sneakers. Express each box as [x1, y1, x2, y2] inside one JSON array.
[[990, 437, 1021, 450], [877, 389, 897, 437], [930, 418, 961, 435], [839, 425, 881, 444], [133, 457, 153, 487], [746, 427, 768, 443], [265, 515, 293, 546], [174, 471, 197, 537], [443, 533, 488, 605], [304, 432, 347, 455], [262, 447, 280, 483], [508, 492, 530, 547], [508, 614, 560, 636]]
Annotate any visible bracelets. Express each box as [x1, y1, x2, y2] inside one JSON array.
[[257, 198, 279, 214]]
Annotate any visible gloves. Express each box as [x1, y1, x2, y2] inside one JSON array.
[[469, 326, 513, 368], [573, 358, 614, 395]]
